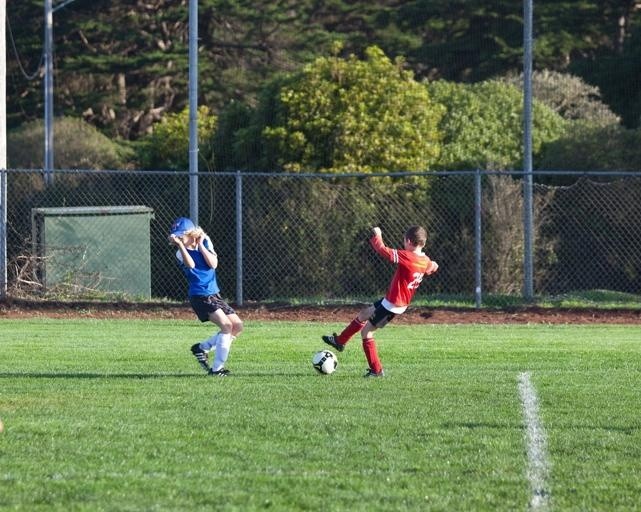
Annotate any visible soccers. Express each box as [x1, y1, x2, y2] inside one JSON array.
[[312, 349, 338, 375]]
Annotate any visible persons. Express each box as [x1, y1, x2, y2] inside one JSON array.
[[321, 225, 440, 377], [168, 217, 244, 377]]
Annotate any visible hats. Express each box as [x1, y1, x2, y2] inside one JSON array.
[[169, 217, 195, 237]]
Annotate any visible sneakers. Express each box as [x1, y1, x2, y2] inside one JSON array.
[[322, 333, 345, 352], [363, 369, 385, 377], [207, 368, 230, 377], [191, 343, 208, 370]]
